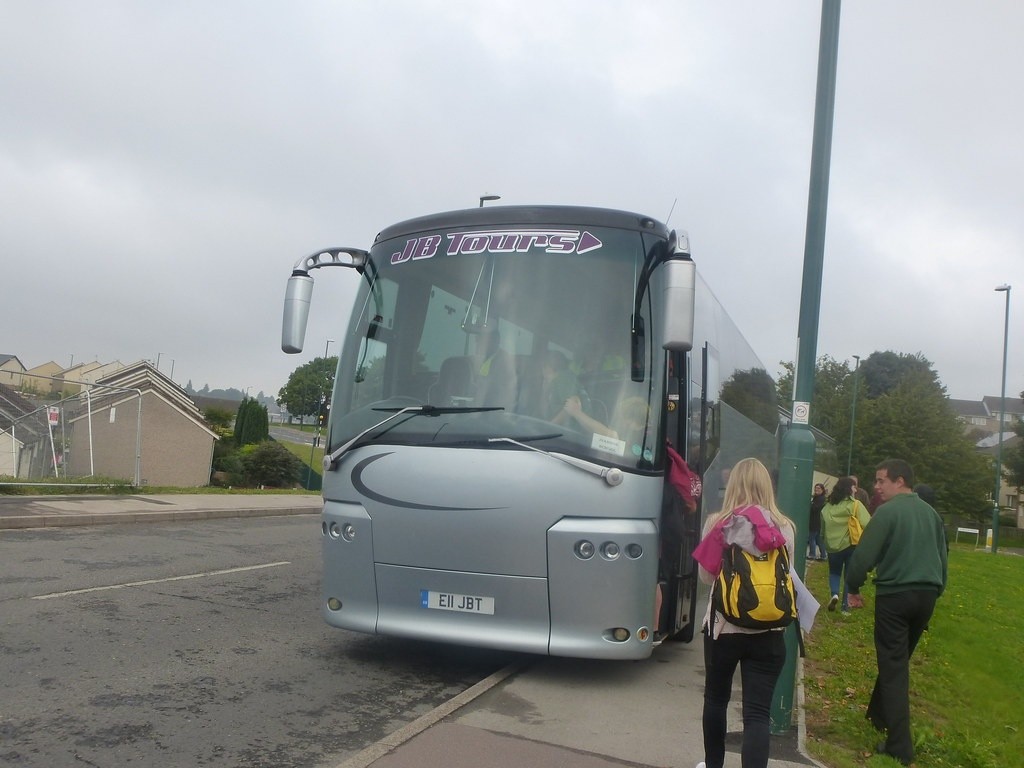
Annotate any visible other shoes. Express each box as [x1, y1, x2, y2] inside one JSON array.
[[816, 557, 827, 562], [841, 611, 851, 617], [923, 626, 928, 633], [877, 743, 909, 766], [827, 594, 839, 612], [807, 556, 816, 560], [652, 632, 662, 646], [696, 762, 706, 768]]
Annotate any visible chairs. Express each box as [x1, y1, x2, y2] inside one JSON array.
[[438, 357, 478, 398], [515, 354, 546, 416]]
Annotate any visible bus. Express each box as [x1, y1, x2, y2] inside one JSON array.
[[779, 403, 841, 544], [278, 201, 788, 662]]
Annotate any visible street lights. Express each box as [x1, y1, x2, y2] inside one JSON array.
[[847, 353, 861, 476], [304, 338, 335, 490], [990, 283, 1013, 552]]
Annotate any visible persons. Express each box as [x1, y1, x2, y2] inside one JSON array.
[[805, 476, 935, 611], [563, 396, 687, 644], [845, 460, 949, 765], [692, 458, 794, 768], [469, 330, 577, 429]]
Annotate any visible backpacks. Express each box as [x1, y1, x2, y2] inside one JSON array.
[[702, 503, 806, 657]]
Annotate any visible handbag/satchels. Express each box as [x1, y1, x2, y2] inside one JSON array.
[[848, 500, 863, 545]]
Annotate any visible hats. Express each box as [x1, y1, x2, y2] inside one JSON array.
[[914, 485, 937, 508]]
